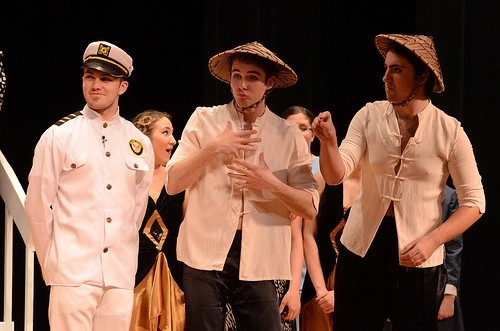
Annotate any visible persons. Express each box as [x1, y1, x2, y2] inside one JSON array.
[[166, 43, 319, 331], [132, 107, 465, 331], [313, 31, 486, 331], [26, 41, 155, 331]]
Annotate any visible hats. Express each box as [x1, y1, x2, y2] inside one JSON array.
[[208, 39, 299, 88], [374, 31, 445, 92], [81, 40, 135, 79]]
